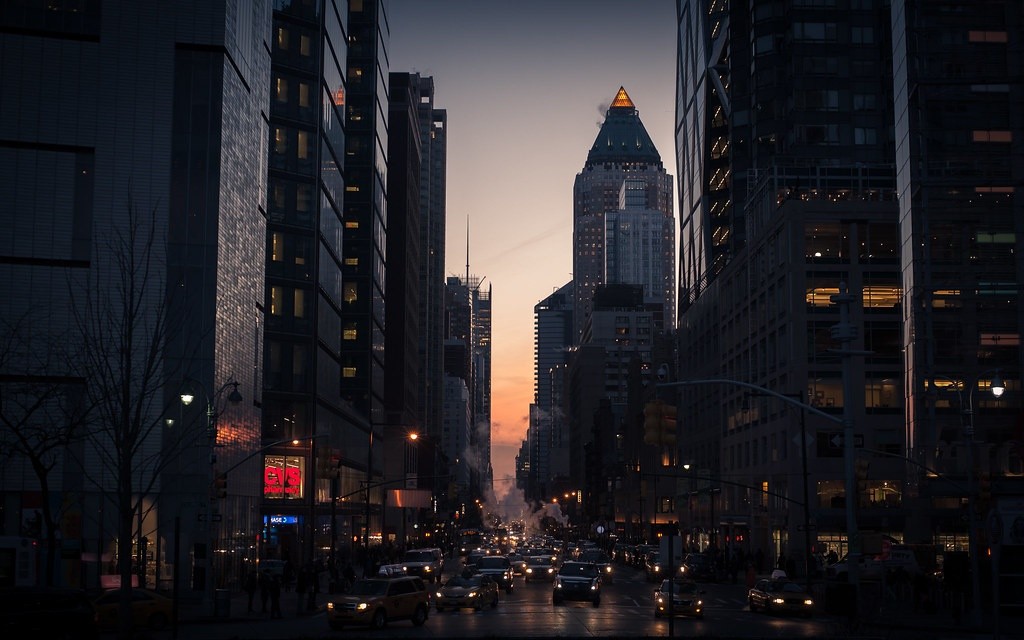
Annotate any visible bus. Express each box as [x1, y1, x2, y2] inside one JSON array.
[[457, 528, 483, 555]]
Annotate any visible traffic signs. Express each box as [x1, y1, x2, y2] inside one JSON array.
[[198, 514, 223, 521], [798, 525, 817, 531]]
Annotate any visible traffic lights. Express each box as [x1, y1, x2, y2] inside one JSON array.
[[643, 400, 678, 446], [449, 480, 466, 503], [215, 473, 227, 499], [316, 448, 342, 482], [853, 456, 871, 480]]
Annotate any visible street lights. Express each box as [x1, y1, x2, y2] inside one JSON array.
[[163, 375, 243, 623], [365, 420, 420, 548], [925, 366, 1007, 635]]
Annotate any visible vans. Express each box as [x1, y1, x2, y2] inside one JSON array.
[[404, 547, 445, 582]]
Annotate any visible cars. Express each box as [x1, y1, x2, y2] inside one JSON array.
[[435, 574, 499, 611], [654, 578, 704, 620], [327, 564, 430, 631], [461, 528, 715, 585], [552, 559, 602, 608], [476, 555, 514, 593], [83, 588, 175, 630], [747, 570, 816, 619]]
